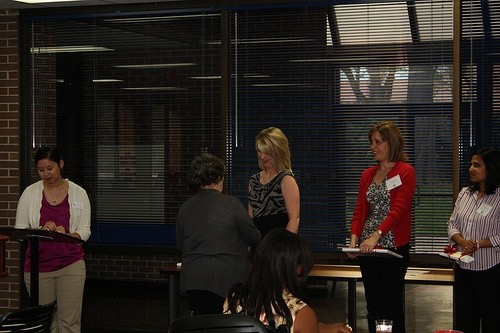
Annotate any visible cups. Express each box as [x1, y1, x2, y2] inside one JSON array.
[[374, 319, 394, 333]]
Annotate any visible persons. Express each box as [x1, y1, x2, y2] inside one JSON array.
[[223, 228, 352, 333], [348, 121, 417, 333], [175, 153, 261, 318], [447, 146, 500, 333], [44, 178, 64, 204], [248, 127, 300, 234], [14, 146, 91, 333]]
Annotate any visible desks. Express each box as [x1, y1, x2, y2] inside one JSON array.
[[159, 264, 454, 333]]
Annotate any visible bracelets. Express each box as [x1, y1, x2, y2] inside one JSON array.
[[476, 240, 479, 250]]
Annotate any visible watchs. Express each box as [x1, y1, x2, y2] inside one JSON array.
[[374, 230, 384, 237]]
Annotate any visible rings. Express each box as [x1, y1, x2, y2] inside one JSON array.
[[365, 248, 369, 250]]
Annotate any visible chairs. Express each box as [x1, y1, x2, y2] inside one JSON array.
[[166, 314, 267, 332]]
